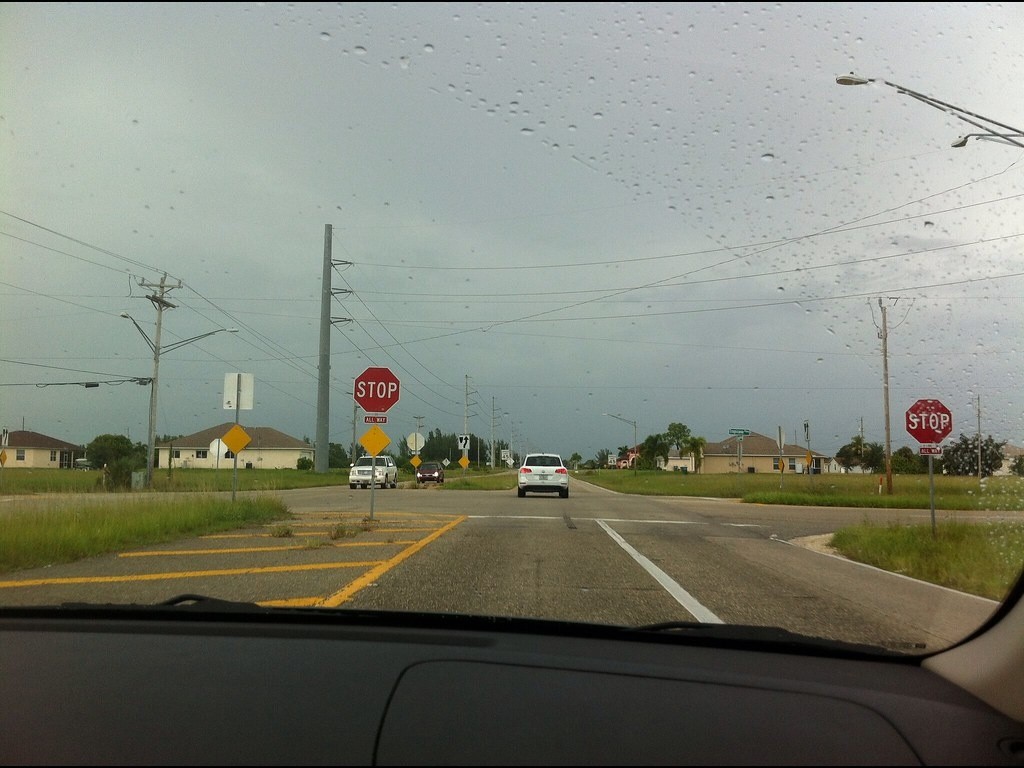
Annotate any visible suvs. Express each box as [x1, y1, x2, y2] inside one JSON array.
[[348, 456, 398, 490]]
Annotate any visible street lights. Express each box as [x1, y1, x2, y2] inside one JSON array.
[[602, 413, 637, 478], [121, 311, 239, 484]]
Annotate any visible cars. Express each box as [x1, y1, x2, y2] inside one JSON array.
[[517, 453, 569, 498], [417, 461, 444, 484]]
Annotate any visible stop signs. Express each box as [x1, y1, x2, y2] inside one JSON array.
[[353, 367, 400, 413], [906, 400, 952, 444]]
